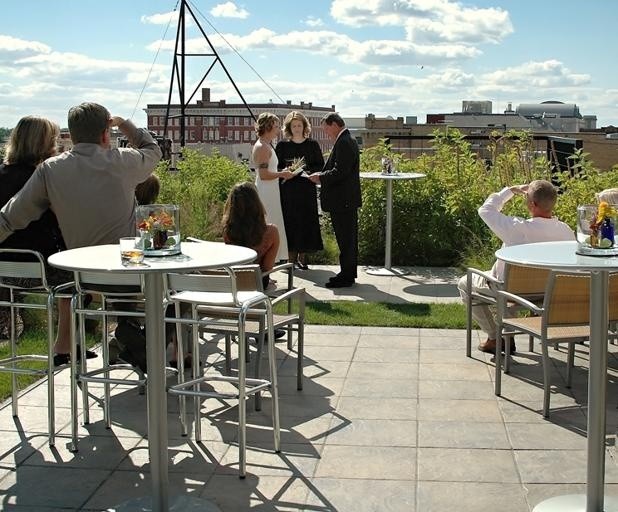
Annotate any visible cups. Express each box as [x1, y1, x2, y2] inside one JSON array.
[[119, 237, 145, 268]]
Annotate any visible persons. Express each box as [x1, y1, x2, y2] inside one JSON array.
[[134, 175, 160, 207], [277, 110, 324, 269], [308, 114, 362, 286], [458, 182, 577, 361], [248, 112, 292, 270], [0, 115, 101, 367], [0, 104, 195, 371], [223, 183, 287, 341]]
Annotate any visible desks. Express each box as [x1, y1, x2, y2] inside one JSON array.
[[495, 240, 618, 512], [360, 173, 426, 276]]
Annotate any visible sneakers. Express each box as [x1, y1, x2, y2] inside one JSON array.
[[255, 330, 285, 343]]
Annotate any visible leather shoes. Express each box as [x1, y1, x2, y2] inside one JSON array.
[[325, 271, 355, 288], [296, 261, 308, 269], [115, 321, 147, 373], [478, 337, 516, 351]]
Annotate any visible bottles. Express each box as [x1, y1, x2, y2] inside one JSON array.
[[600, 216, 615, 248]]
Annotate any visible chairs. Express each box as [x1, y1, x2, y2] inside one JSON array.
[[495, 270, 592, 419], [607, 271, 618, 339], [466, 263, 559, 374]]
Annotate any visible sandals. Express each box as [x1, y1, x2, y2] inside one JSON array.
[[54, 344, 98, 367]]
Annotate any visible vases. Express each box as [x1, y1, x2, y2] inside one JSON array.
[[600, 218, 615, 245], [158, 231, 168, 244]]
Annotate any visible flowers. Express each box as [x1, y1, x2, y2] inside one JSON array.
[[596, 202, 618, 226], [138, 210, 175, 243]]
